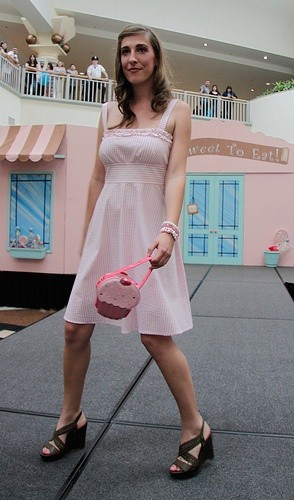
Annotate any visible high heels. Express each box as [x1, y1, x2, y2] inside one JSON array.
[[168, 417, 215, 481], [39, 410, 87, 463]]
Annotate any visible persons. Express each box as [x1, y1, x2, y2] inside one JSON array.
[[0, 41, 108, 103], [193, 80, 237, 120], [40, 24, 215, 480]]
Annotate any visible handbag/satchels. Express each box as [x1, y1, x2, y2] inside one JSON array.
[[94, 256, 157, 320]]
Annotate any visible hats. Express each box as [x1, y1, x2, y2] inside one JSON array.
[[92, 56, 100, 60]]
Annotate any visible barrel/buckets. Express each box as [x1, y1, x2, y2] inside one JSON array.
[[264, 250, 280, 267]]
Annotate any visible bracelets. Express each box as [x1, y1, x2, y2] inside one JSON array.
[[160, 221, 179, 241]]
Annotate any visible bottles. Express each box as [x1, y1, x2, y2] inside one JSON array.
[[15, 226, 21, 246], [28, 228, 34, 245]]
